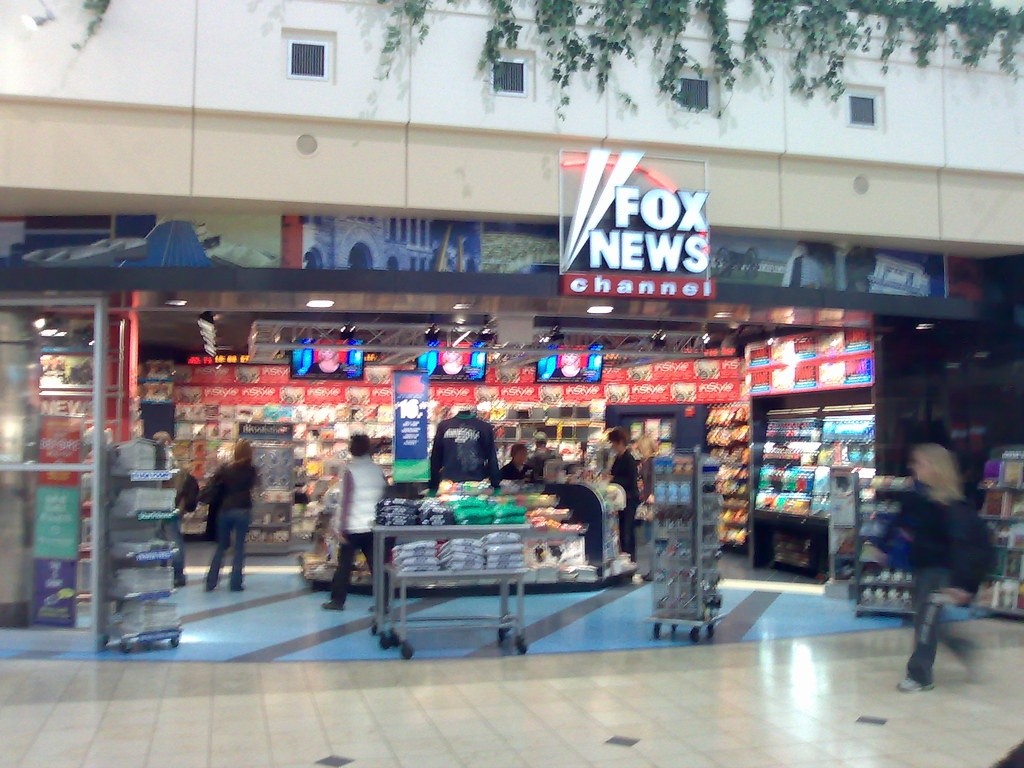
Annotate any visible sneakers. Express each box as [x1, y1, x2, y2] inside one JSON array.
[[898, 676, 934, 691]]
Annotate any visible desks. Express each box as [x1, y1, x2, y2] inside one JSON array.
[[367, 519, 534, 659]]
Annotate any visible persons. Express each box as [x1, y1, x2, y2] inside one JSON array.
[[202, 437, 257, 592], [430, 411, 503, 499], [874, 444, 987, 693], [322, 433, 396, 613], [499, 433, 556, 483], [602, 430, 641, 562], [153, 431, 187, 587]]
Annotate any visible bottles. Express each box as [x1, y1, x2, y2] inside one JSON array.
[[755, 421, 874, 521], [991, 579, 1024, 609]]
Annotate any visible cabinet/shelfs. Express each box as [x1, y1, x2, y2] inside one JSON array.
[[36, 317, 126, 602], [104, 439, 184, 653], [975, 447, 1024, 620], [649, 445, 726, 643], [852, 472, 916, 618], [754, 405, 876, 528], [234, 421, 294, 555]]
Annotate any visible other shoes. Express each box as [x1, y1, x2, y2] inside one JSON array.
[[322, 601, 344, 610]]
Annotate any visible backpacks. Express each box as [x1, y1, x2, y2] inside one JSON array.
[[176, 471, 200, 513]]
